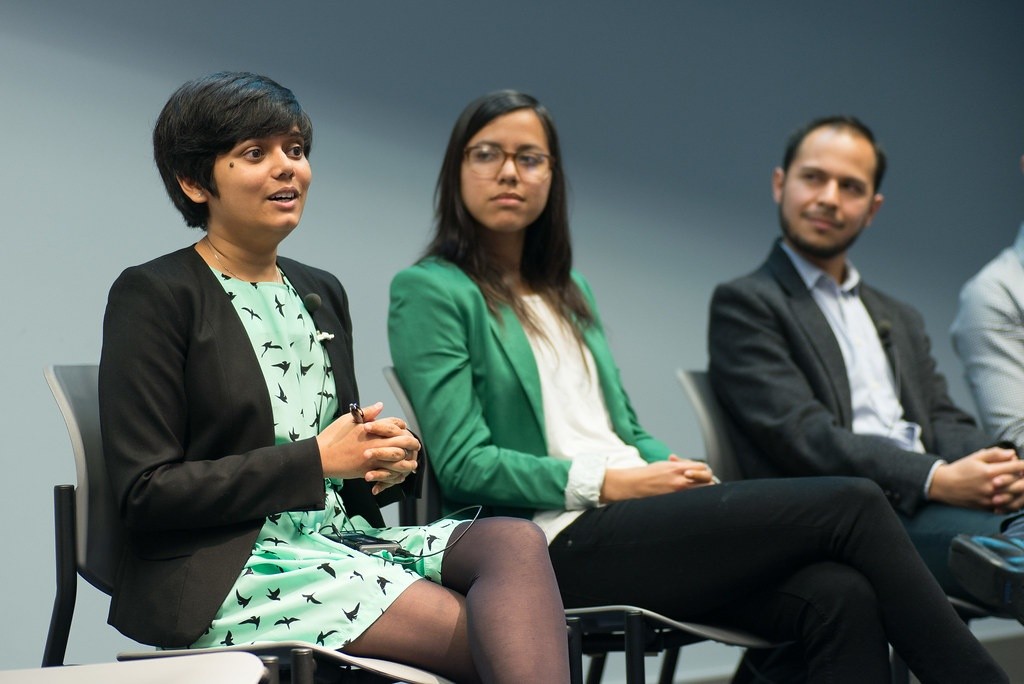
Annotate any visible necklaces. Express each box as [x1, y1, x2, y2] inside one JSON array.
[[204, 238, 280, 283]]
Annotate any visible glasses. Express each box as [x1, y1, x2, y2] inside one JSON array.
[[464, 145, 555, 185]]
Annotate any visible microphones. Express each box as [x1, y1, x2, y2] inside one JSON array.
[[303, 294, 321, 312], [878, 320, 893, 349]]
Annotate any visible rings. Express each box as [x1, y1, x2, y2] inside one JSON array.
[[404, 448, 410, 460]]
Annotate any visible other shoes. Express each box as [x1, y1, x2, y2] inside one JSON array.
[[949, 535, 1024, 619]]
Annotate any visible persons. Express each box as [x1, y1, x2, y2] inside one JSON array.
[[948, 231, 1024, 452], [708, 114, 1024, 624], [98, 72, 570, 684], [388, 88, 1009, 684]]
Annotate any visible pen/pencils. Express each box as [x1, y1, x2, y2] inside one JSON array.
[[348, 402, 365, 424]]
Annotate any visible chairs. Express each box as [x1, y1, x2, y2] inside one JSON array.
[[380, 366, 794, 684], [673, 366, 992, 684], [42, 364, 455, 684]]
